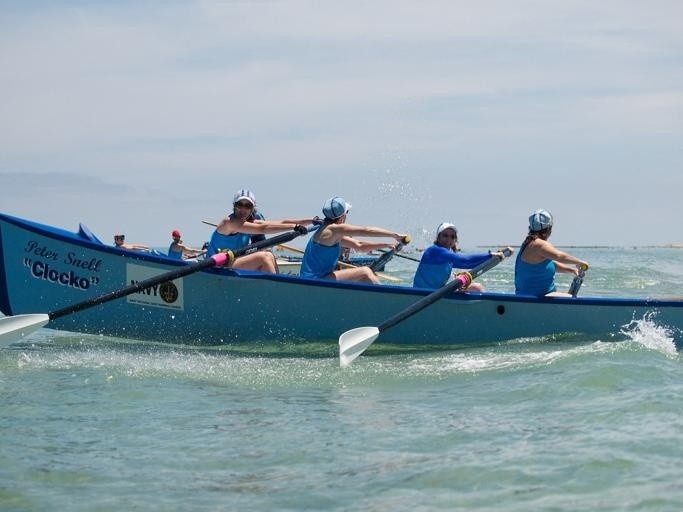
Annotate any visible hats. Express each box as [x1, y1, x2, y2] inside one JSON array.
[[528, 208, 554, 231], [437, 222, 459, 237], [113, 235, 124, 240], [322, 197, 353, 220], [233, 187, 257, 208], [172, 230, 180, 236]]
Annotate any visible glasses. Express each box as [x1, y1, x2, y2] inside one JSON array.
[[441, 234, 457, 238], [233, 201, 253, 208]]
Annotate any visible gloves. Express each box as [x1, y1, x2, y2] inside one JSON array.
[[293, 224, 307, 234], [312, 215, 325, 226]]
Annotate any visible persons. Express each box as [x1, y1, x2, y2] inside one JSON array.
[[515, 208, 589, 298], [112, 190, 325, 274], [301, 196, 408, 285], [414, 221, 514, 292]]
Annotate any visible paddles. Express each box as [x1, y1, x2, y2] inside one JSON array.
[[338, 251, 511, 366], [0, 223, 321, 347]]
